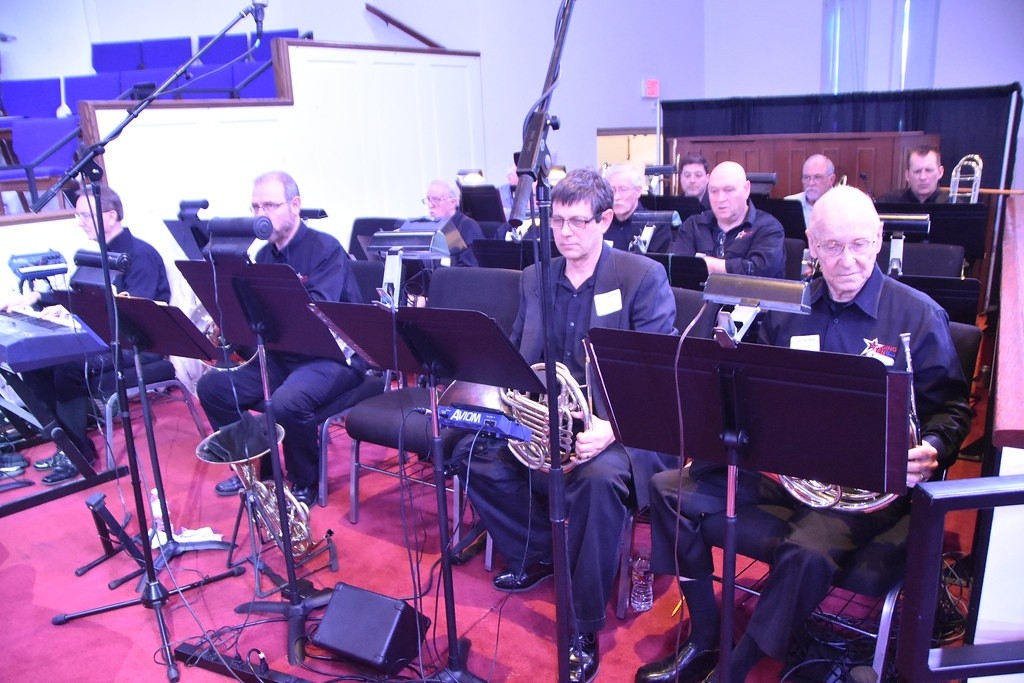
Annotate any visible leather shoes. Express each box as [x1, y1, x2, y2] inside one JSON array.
[[566, 627, 599, 683], [493, 557, 556, 592], [635, 634, 736, 683], [215, 462, 275, 496], [286, 481, 321, 515], [33, 450, 67, 470], [43, 453, 95, 486]]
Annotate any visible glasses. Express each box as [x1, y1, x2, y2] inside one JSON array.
[[75, 207, 116, 224], [548, 208, 608, 230], [810, 229, 879, 256], [801, 174, 831, 183], [716, 232, 726, 257], [422, 196, 455, 207], [250, 197, 294, 215]]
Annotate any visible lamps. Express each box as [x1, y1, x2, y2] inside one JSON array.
[[73, 162, 932, 352]]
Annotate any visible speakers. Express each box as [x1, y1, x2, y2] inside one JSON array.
[[314, 582, 431, 676]]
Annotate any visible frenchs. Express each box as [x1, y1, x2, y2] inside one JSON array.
[[434, 362, 592, 475], [776, 332, 924, 515], [188, 301, 261, 372], [194, 422, 334, 563]]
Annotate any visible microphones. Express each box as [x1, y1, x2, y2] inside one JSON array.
[[252, 0, 269, 38]]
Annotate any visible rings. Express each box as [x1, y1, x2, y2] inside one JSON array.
[[919, 473, 924, 480]]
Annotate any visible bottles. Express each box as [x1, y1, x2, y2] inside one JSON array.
[[630, 547, 654, 611], [151, 487, 174, 535]]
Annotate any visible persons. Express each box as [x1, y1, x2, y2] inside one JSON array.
[[454, 168, 680, 683], [680, 153, 711, 209], [602, 163, 672, 253], [878, 146, 963, 203], [499, 164, 520, 218], [195, 170, 365, 515], [666, 162, 787, 278], [633, 183, 971, 683], [0, 184, 171, 486], [784, 154, 836, 260], [422, 178, 485, 267]]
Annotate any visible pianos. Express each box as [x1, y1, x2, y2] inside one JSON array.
[[0, 304, 128, 520]]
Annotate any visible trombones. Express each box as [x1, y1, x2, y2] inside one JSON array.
[[808, 174, 849, 277], [946, 152, 984, 204], [672, 138, 680, 196]]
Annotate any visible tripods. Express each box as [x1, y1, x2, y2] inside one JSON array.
[[33, 1, 551, 683]]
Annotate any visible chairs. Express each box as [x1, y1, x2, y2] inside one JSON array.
[[85, 219, 984, 682], [0, 26, 301, 216]]
